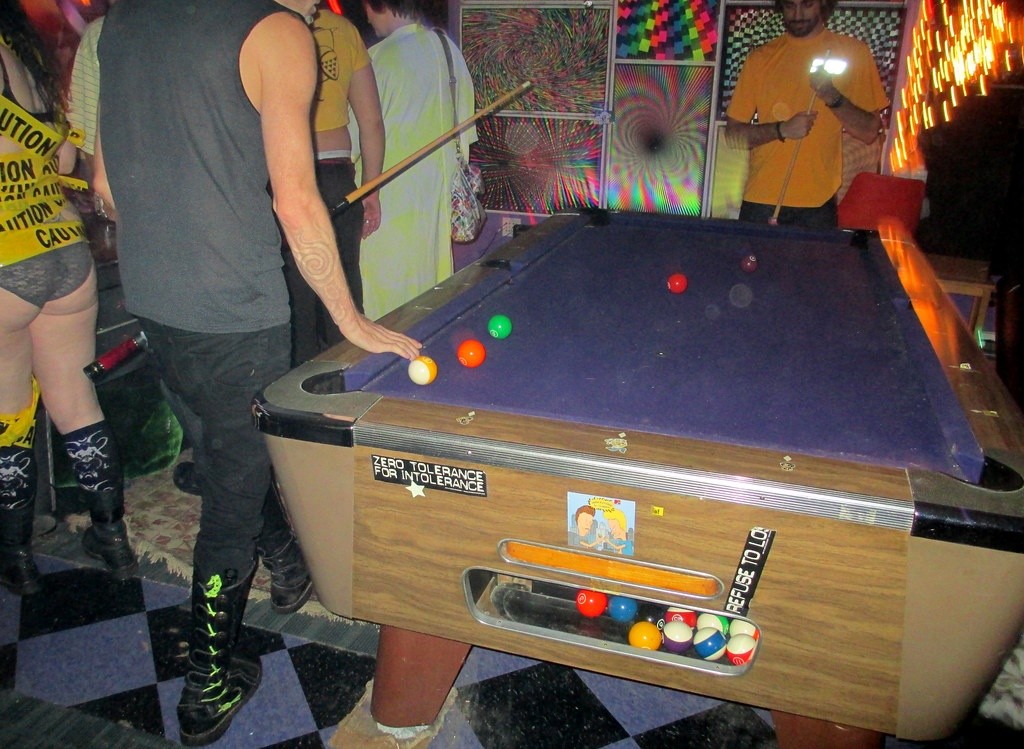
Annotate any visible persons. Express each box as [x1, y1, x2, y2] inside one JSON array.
[[96, 0, 422, 746], [66, 0, 477, 493], [0, 0, 138, 592], [725, 0, 890, 231]]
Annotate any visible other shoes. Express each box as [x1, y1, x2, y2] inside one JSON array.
[[172, 462, 203, 495]]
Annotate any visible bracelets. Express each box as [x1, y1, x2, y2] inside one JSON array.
[[776, 121, 785, 141]]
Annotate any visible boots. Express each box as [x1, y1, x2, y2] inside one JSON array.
[[0, 491, 42, 593], [80, 477, 134, 572], [258, 529, 314, 616], [176, 562, 264, 747]]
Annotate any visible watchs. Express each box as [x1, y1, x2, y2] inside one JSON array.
[[826, 94, 844, 108]]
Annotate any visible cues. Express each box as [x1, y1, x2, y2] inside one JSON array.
[[771, 47, 831, 222], [81, 80, 533, 382]]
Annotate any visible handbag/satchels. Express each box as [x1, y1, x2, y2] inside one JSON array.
[[451, 158, 486, 244]]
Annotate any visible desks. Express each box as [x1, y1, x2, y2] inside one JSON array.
[[253, 209, 1023, 748]]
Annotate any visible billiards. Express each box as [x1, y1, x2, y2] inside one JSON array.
[[407, 355, 438, 386], [741, 256, 758, 273], [457, 339, 487, 368], [667, 273, 688, 294], [575, 589, 760, 666], [488, 314, 513, 339]]
